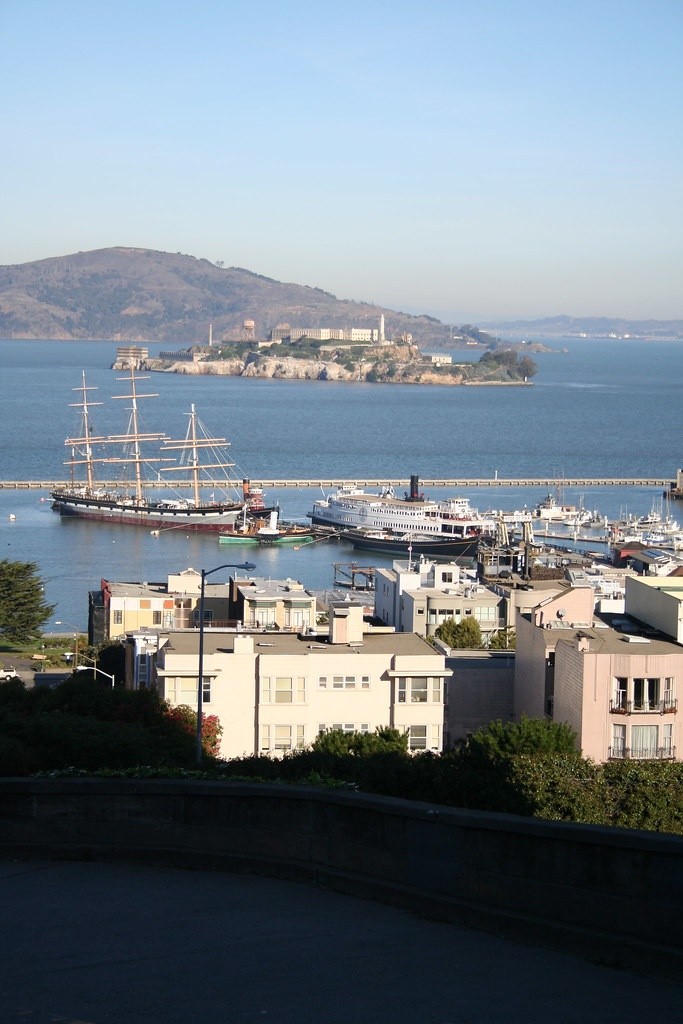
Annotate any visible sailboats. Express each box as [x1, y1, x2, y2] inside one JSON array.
[[50, 363, 280, 533], [605, 485, 683, 549]]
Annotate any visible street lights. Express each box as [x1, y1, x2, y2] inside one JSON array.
[[77, 666, 114, 689], [196, 561, 256, 762], [55, 621, 79, 667], [64, 652, 96, 680]]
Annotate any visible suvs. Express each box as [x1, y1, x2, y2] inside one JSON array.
[[0, 668, 17, 681]]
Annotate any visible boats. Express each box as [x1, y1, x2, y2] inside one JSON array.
[[563, 496, 607, 528], [306, 475, 507, 540], [351, 534, 478, 562], [531, 470, 577, 520]]
